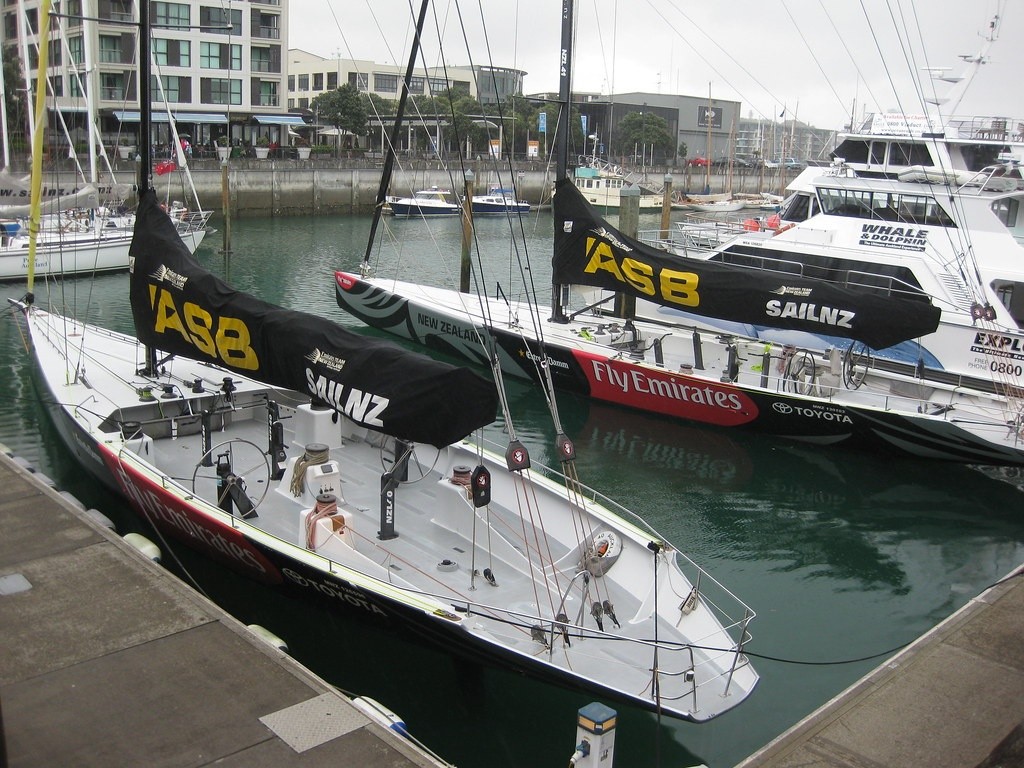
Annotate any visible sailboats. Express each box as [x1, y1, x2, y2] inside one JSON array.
[[0, 1, 762, 723], [1, 1, 1024, 469]]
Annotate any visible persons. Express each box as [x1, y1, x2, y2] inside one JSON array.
[[173, 139, 192, 159]]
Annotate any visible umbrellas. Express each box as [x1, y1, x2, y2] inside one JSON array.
[[179, 134, 190, 138]]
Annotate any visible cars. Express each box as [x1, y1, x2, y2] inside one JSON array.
[[753, 159, 777, 170], [722, 156, 751, 169], [687, 157, 714, 168]]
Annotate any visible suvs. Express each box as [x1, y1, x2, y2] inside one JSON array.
[[775, 157, 801, 170]]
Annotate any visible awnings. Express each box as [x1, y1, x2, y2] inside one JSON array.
[[112, 111, 230, 124], [254, 115, 306, 126]]
